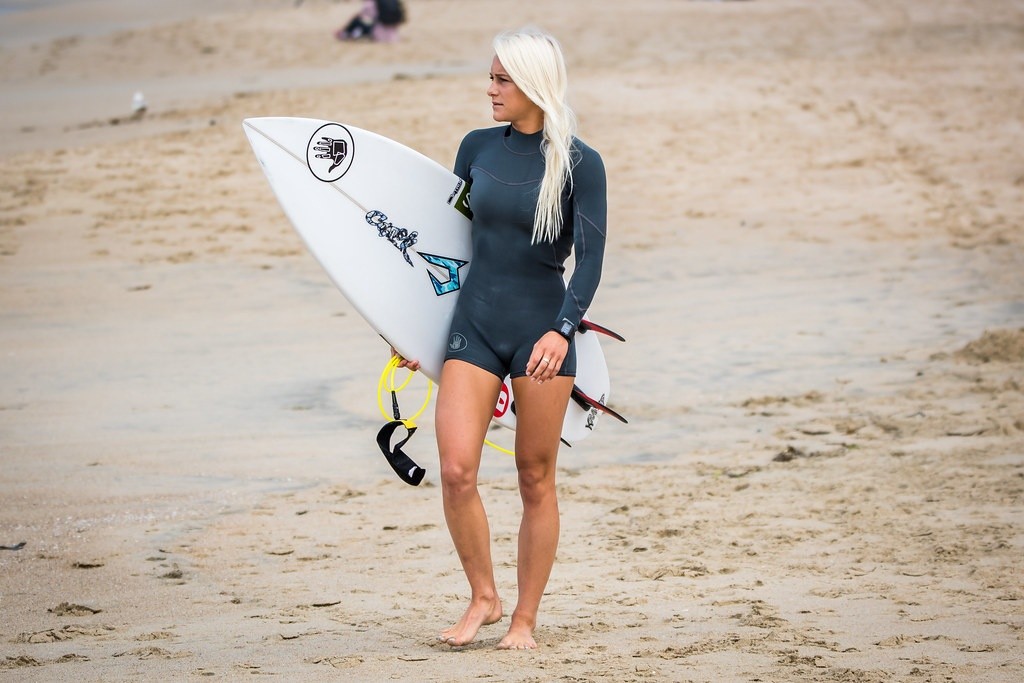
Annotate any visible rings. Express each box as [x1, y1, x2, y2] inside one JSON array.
[[542, 358, 549, 364]]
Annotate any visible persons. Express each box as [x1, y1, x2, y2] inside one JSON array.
[[335, 0, 404, 41], [391, 35, 607, 651]]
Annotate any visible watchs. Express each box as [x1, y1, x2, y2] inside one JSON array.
[[550, 320, 574, 344]]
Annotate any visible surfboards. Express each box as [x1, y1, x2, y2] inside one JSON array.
[[242, 116, 628, 448]]
